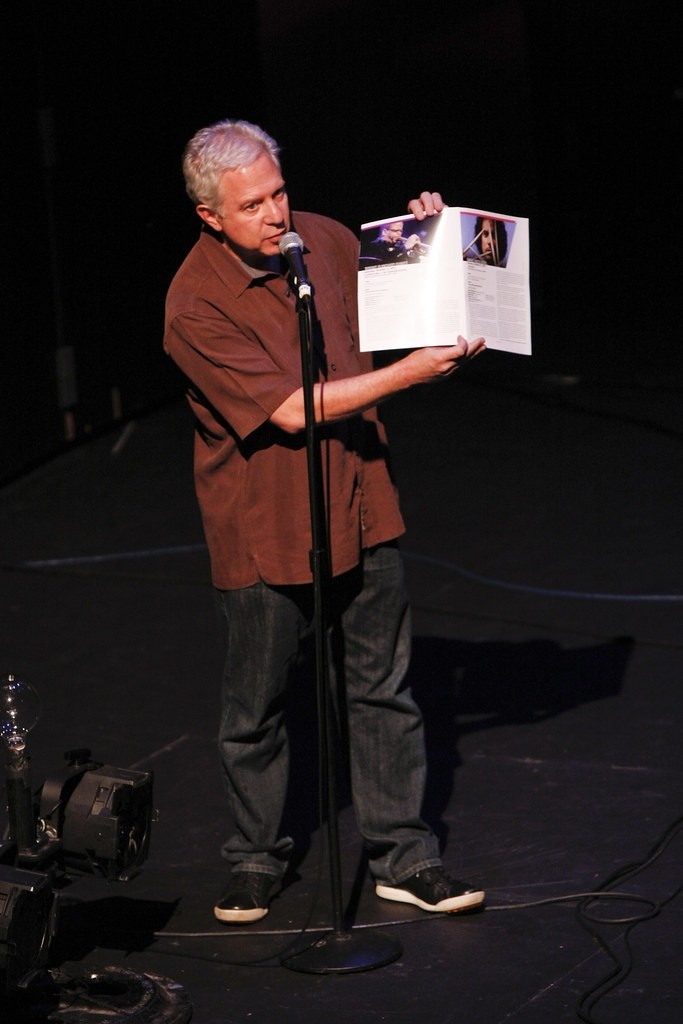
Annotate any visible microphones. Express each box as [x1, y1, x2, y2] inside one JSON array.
[[280, 231, 312, 304]]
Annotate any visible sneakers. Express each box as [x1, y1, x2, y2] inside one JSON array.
[[374, 866, 484, 913], [214, 873, 284, 921]]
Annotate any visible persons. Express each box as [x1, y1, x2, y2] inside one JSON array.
[[166, 119, 485, 923], [359, 221, 426, 272], [474, 217, 508, 270]]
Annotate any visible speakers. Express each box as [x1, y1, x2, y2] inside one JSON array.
[[39, 767, 153, 864]]
